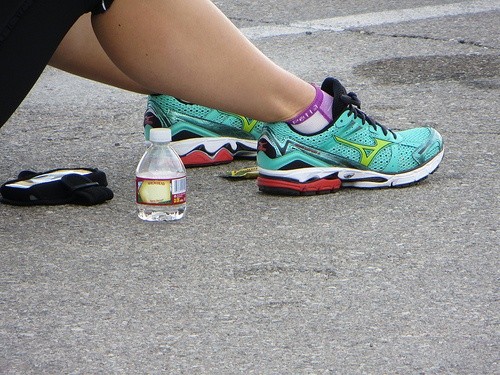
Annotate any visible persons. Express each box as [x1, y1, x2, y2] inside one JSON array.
[[0, 1, 447, 197]]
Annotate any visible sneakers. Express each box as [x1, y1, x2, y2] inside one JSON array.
[[143, 96, 265, 167], [256, 74, 446, 192]]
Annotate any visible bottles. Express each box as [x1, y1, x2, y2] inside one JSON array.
[[134, 128, 186, 222]]
